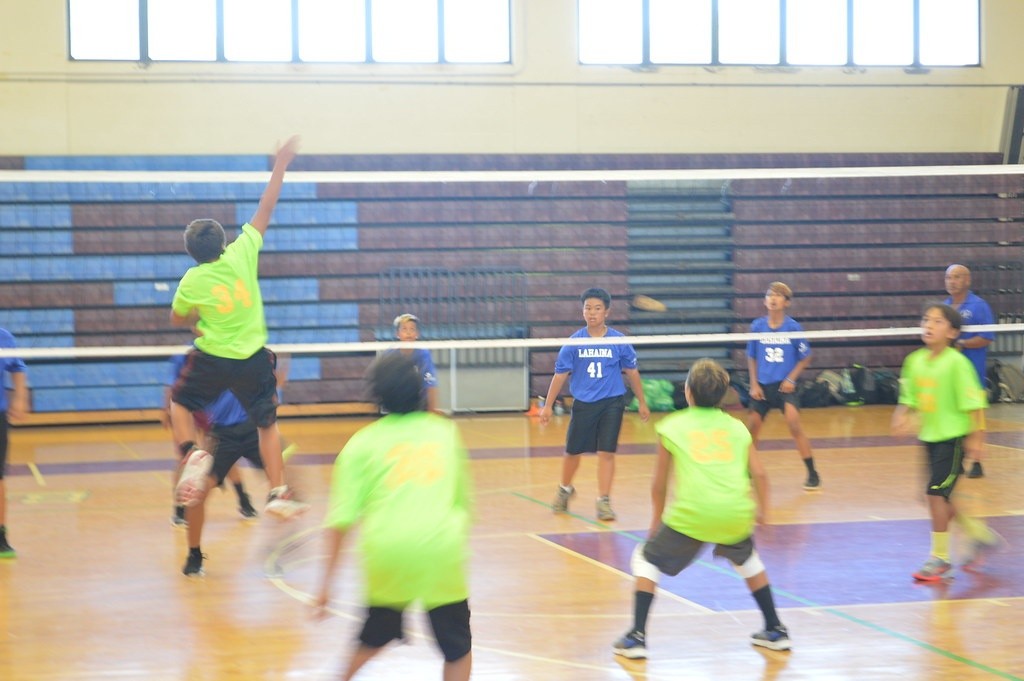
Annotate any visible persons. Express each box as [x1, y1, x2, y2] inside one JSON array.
[[169, 343, 292, 580], [382, 312, 449, 416], [165, 133, 312, 527], [611, 358, 794, 659], [0, 327, 29, 561], [308, 350, 485, 681], [746, 281, 822, 490], [887, 302, 1013, 583], [539, 288, 651, 521], [941, 263, 998, 478]]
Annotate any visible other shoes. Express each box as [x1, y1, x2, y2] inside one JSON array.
[[969, 465, 982, 478], [0, 527, 14, 557]]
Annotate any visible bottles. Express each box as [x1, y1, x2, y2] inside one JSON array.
[[554, 401, 563, 426], [538, 397, 544, 413]]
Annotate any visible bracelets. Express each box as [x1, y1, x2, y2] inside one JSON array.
[[784, 377, 798, 385]]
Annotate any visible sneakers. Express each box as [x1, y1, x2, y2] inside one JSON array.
[[597, 498, 615, 521], [912, 556, 950, 582], [752, 623, 791, 650], [554, 484, 573, 512], [613, 632, 646, 659], [173, 450, 215, 506], [171, 508, 185, 526], [964, 537, 1008, 572], [267, 490, 300, 516], [805, 472, 819, 489], [237, 494, 257, 518], [182, 552, 207, 577]]
